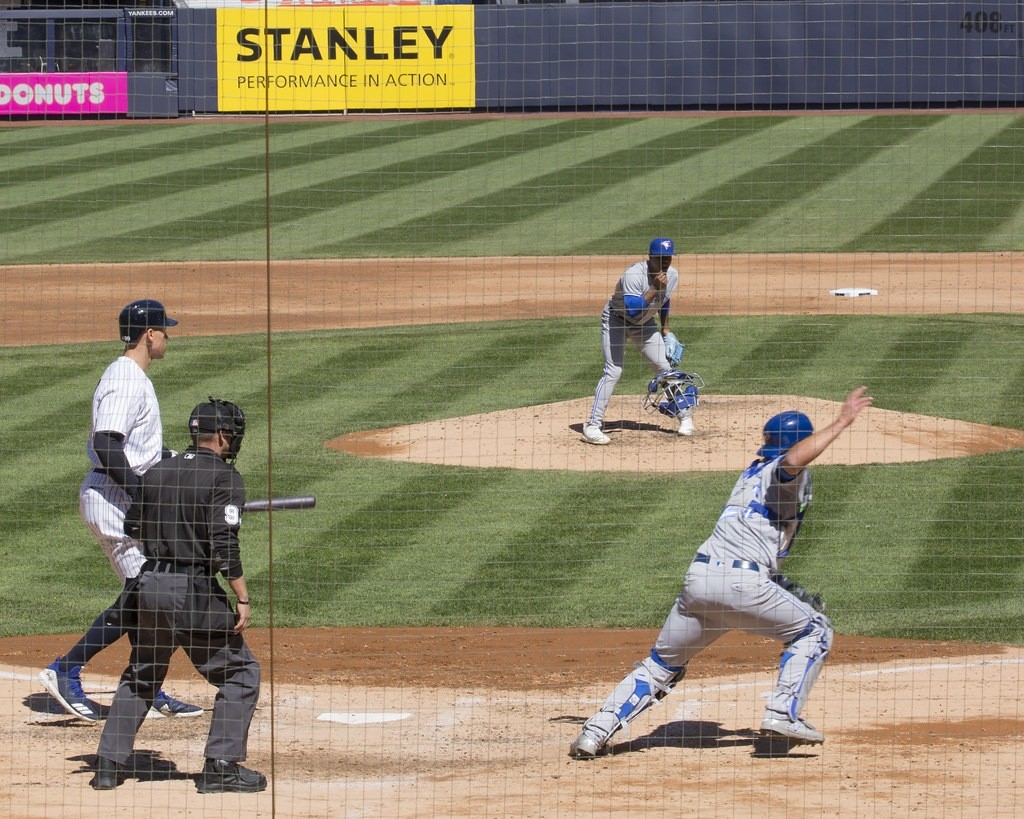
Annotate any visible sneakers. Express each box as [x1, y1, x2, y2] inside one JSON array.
[[145, 691, 204, 719], [575, 735, 610, 758], [197, 759, 267, 794], [761, 711, 826, 747], [677, 417, 693, 436], [92, 757, 125, 790], [39, 656, 99, 722], [580, 422, 611, 445]]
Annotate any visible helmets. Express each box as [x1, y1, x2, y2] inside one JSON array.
[[119, 299, 177, 343], [757, 411, 814, 457]]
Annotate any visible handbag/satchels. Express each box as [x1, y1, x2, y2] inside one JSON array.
[[107, 573, 140, 631], [177, 566, 238, 637]]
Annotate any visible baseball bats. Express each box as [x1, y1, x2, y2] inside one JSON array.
[[241, 496, 318, 513]]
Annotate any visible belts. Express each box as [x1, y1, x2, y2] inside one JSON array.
[[92, 467, 109, 475], [692, 554, 758, 571], [610, 306, 633, 324], [148, 560, 189, 574]]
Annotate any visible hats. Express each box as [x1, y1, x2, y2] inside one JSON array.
[[189, 402, 233, 431], [648, 238, 674, 256]]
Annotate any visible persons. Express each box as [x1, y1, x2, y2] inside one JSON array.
[[581, 240, 694, 445], [93, 395, 268, 793], [570, 385, 876, 757], [40, 300, 207, 722]]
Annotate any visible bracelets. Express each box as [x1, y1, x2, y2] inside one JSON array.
[[238, 599, 249, 604]]
